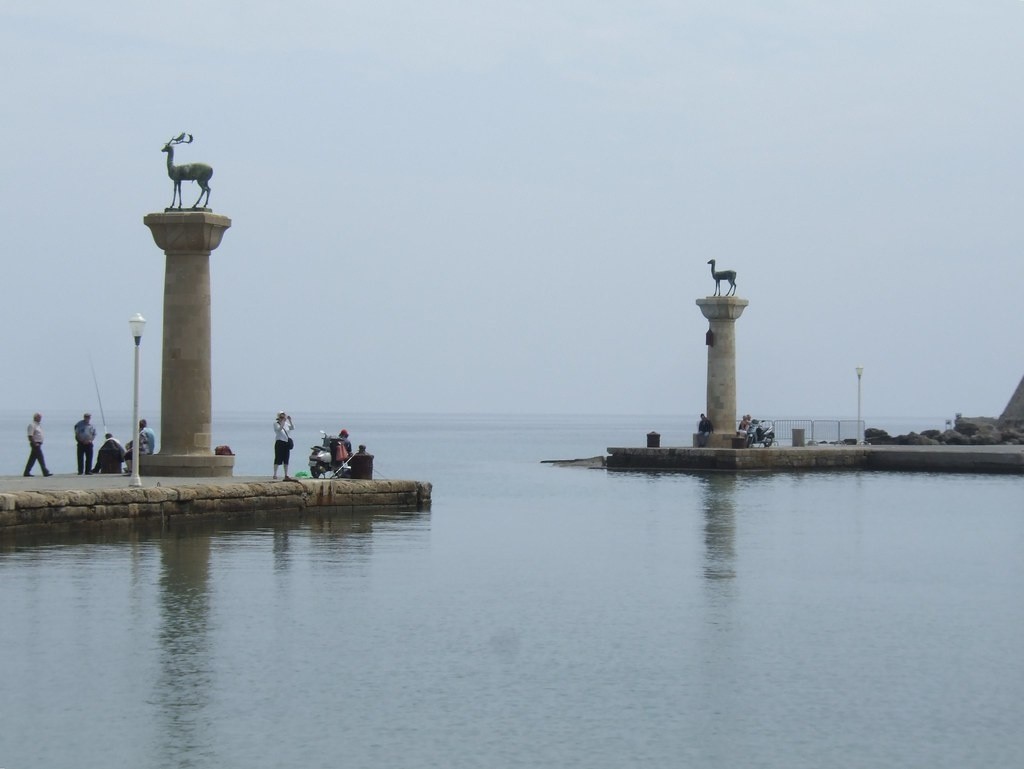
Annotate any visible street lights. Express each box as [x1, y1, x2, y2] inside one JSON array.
[[855, 367, 864, 445], [128, 313, 147, 487]]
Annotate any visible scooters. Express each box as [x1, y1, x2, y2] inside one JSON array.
[[308, 430, 342, 479], [745, 418, 776, 448]]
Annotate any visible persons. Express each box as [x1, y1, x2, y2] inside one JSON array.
[[696, 414, 714, 447], [123, 419, 154, 475], [746, 415, 752, 430], [337, 430, 352, 457], [91, 433, 125, 472], [738, 415, 747, 430], [22, 412, 53, 477], [75, 413, 97, 475], [273, 411, 295, 480]]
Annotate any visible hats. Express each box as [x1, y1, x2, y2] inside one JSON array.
[[340, 429, 349, 436], [84, 413, 91, 417]]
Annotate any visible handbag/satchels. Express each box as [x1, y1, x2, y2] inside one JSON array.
[[336, 442, 348, 461], [288, 438, 294, 450]]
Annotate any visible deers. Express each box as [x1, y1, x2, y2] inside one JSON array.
[[162, 132, 213, 209]]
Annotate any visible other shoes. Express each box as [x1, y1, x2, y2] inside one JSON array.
[[24, 473, 34, 477], [78, 471, 83, 475], [85, 471, 92, 475], [90, 469, 99, 473], [272, 476, 277, 480], [44, 473, 53, 477], [122, 468, 132, 476], [285, 475, 292, 480]]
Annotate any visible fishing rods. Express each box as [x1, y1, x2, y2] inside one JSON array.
[[91, 366, 108, 434], [373, 469, 387, 479]]
